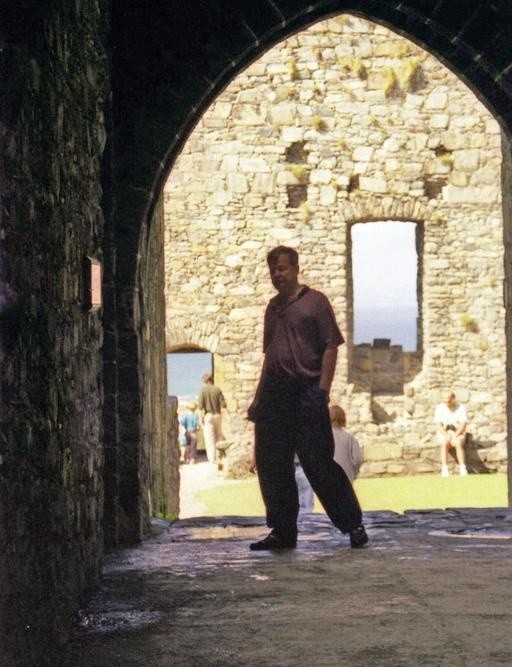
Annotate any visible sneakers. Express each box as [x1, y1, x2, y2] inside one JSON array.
[[179, 455, 196, 464], [459, 463, 468, 475], [441, 465, 449, 476]]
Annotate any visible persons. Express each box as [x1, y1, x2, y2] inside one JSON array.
[[179, 401, 200, 464], [326, 403, 364, 494], [432, 388, 469, 477], [244, 244, 371, 549], [197, 372, 228, 463]]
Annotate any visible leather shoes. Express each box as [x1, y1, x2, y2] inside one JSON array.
[[349, 523, 369, 548], [249, 533, 296, 551]]
